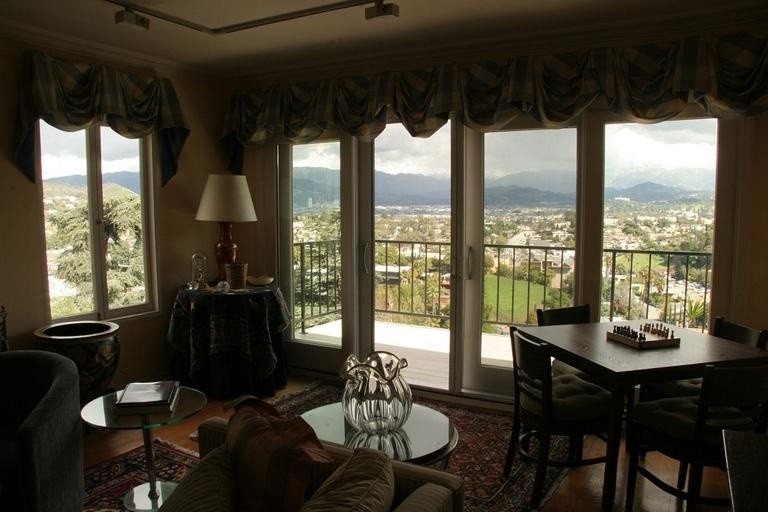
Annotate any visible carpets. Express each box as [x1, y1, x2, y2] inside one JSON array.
[[190, 378, 589, 511], [83, 437, 200, 512]]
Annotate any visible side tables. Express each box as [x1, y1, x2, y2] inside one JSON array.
[[80, 386, 208, 511]]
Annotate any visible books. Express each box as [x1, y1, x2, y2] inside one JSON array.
[[115, 382, 179, 414]]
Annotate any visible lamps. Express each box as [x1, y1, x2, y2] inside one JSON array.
[[193, 170, 259, 285], [365, 4, 399, 22], [115, 12, 150, 34]]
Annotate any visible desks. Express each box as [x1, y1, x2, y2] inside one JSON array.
[[511, 319, 767, 512], [164, 283, 294, 398]]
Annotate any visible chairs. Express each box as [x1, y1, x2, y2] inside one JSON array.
[[625, 364, 768, 512], [0, 348, 84, 511], [502, 325, 624, 508], [537, 305, 650, 464], [642, 317, 761, 491]]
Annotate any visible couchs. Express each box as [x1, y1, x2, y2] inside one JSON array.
[[159, 417, 464, 512]]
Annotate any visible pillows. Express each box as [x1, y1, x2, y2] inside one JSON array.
[[223, 394, 334, 512], [301, 449, 395, 512], [158, 444, 235, 512]]
